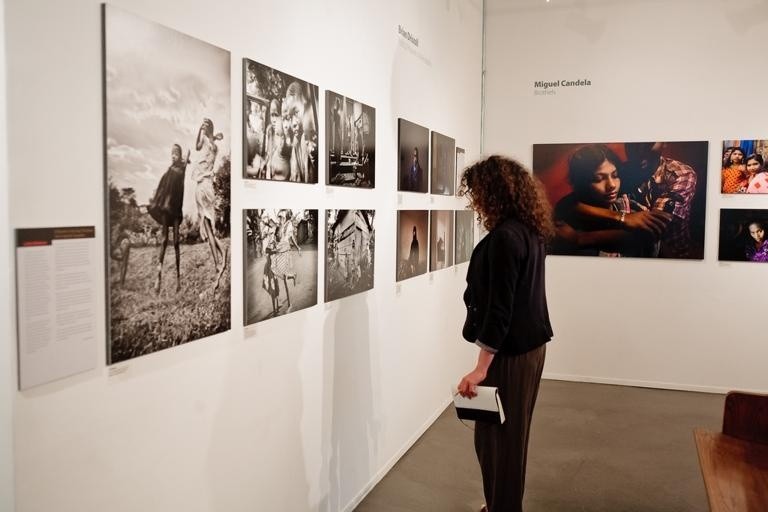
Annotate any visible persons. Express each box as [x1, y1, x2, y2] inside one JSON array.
[[458, 152, 559, 512], [722, 140, 768, 194], [260, 81, 318, 184], [259, 210, 282, 316], [355, 126, 363, 164], [746, 222, 768, 262], [409, 226, 419, 274], [146, 143, 186, 293], [550, 143, 698, 257], [333, 98, 342, 176], [270, 209, 302, 314], [409, 147, 422, 191], [185, 116, 229, 275], [553, 143, 661, 259]]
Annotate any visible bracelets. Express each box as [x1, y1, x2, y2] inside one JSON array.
[[619, 211, 628, 225]]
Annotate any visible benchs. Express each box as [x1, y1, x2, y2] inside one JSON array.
[[691, 385, 768, 512]]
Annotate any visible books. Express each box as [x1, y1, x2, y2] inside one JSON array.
[[450, 383, 506, 425]]
